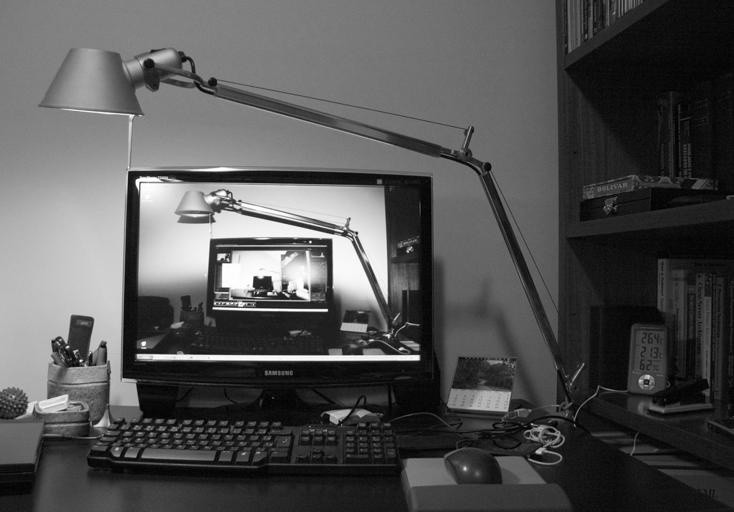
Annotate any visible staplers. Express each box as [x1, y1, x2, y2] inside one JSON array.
[[648, 379, 714, 414]]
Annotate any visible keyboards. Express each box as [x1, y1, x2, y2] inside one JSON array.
[[191, 327, 328, 355], [88, 417, 401, 476]]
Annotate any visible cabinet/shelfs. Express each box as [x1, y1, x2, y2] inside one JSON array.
[[556, 0, 734, 473]]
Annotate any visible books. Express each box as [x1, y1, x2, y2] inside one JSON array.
[[579, 75, 734, 200], [567, 0, 642, 51], [657, 259, 734, 402]]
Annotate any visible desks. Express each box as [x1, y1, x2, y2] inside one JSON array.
[[0, 402, 733, 511]]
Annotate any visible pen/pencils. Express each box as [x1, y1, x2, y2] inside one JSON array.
[[51, 336, 107, 367]]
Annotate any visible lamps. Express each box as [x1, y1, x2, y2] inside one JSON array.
[[36, 41, 582, 430], [174, 190, 390, 331]]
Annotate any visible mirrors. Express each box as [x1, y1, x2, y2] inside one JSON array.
[[121, 168, 436, 385]]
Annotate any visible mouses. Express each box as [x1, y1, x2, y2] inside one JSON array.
[[342, 342, 362, 354], [444, 447, 502, 484]]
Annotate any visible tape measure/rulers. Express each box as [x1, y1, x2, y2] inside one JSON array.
[[65, 312, 95, 367]]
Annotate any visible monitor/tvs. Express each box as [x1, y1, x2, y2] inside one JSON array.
[[205, 239, 333, 327], [120, 170, 434, 426]]
